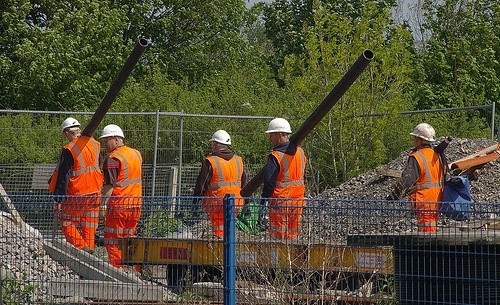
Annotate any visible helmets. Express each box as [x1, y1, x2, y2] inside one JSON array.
[[265, 118, 292, 134], [60, 117, 80, 133], [99, 124, 125, 139], [208, 130, 231, 145], [409, 123, 436, 141]]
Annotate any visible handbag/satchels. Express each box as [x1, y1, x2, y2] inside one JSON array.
[[440, 168, 471, 221]]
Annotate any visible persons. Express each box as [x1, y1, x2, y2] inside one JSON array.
[[193, 130, 251, 238], [100, 124, 142, 273], [385, 123, 446, 232], [264, 118, 306, 239], [49, 118, 104, 256]]
